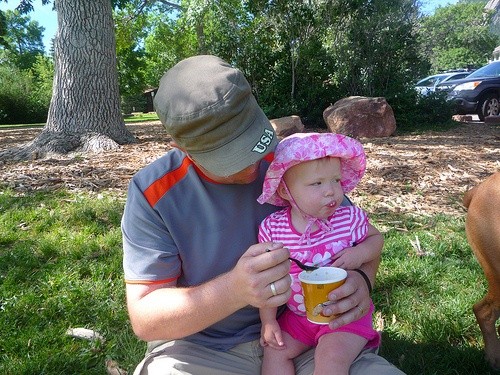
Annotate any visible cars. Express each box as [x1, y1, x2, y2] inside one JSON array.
[[435, 60, 500, 122], [414, 72, 470, 95]]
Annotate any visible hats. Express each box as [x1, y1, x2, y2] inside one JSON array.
[[254, 130, 366, 206], [154, 54, 276, 181]]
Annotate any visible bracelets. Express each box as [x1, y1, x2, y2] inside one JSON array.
[[352, 269, 373, 293]]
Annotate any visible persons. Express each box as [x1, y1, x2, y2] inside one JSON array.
[[121, 55, 405, 374], [258, 132, 384, 375]]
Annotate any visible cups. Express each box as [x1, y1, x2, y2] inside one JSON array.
[[298, 266, 347, 325]]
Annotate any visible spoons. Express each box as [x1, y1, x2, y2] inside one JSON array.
[[265, 249, 318, 271]]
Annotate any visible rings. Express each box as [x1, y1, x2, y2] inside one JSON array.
[[270, 282, 278, 296]]
